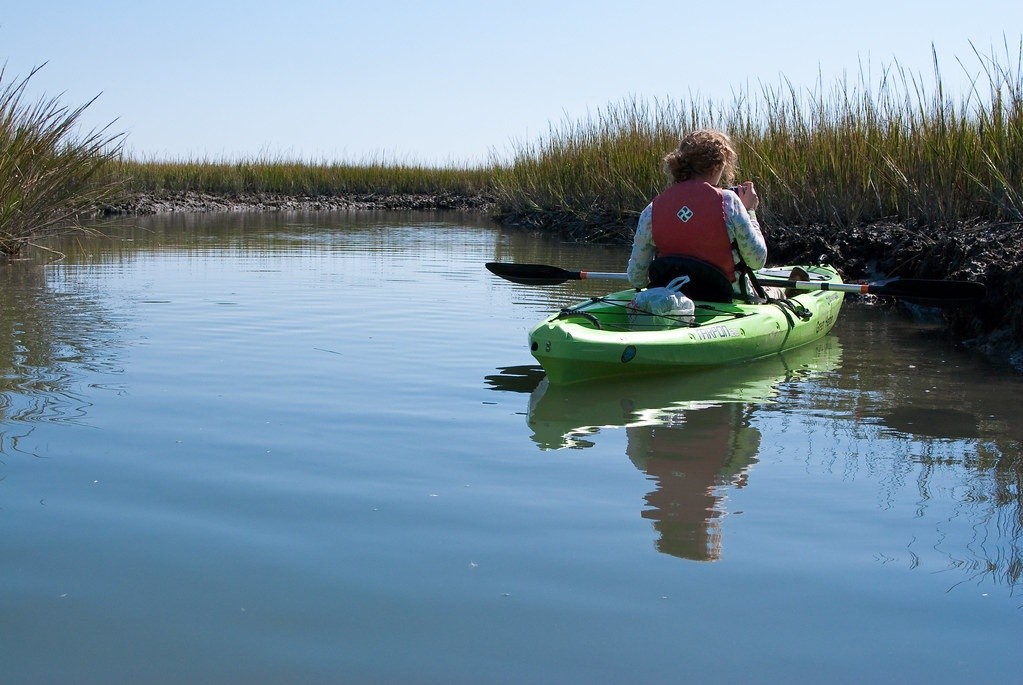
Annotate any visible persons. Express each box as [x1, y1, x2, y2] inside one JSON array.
[[627, 130, 809, 305], [625, 402, 763, 563]]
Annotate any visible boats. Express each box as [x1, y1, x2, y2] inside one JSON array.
[[486, 260, 988, 389]]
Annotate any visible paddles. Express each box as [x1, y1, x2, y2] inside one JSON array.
[[485, 260, 875, 294]]
